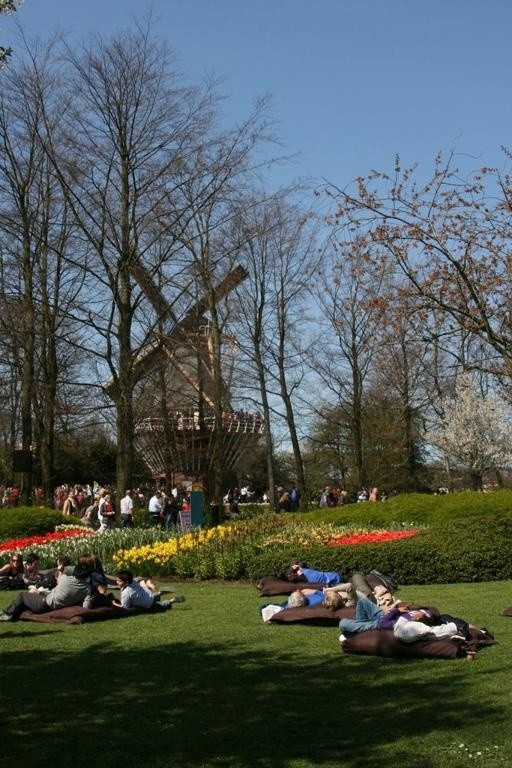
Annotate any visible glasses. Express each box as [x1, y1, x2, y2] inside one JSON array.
[[13, 559, 20, 563]]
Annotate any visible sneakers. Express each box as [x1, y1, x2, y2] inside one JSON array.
[[0, 613, 13, 622], [173, 596, 185, 603], [371, 569, 398, 589], [159, 588, 175, 594]]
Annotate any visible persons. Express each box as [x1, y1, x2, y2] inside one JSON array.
[[0, 552, 185, 621], [278, 564, 469, 646], [433, 490, 440, 495], [440, 490, 447, 495], [210, 482, 397, 522], [1, 483, 191, 533]]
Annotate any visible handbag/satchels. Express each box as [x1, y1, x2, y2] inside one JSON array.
[[441, 614, 469, 638], [36, 572, 57, 590]]
